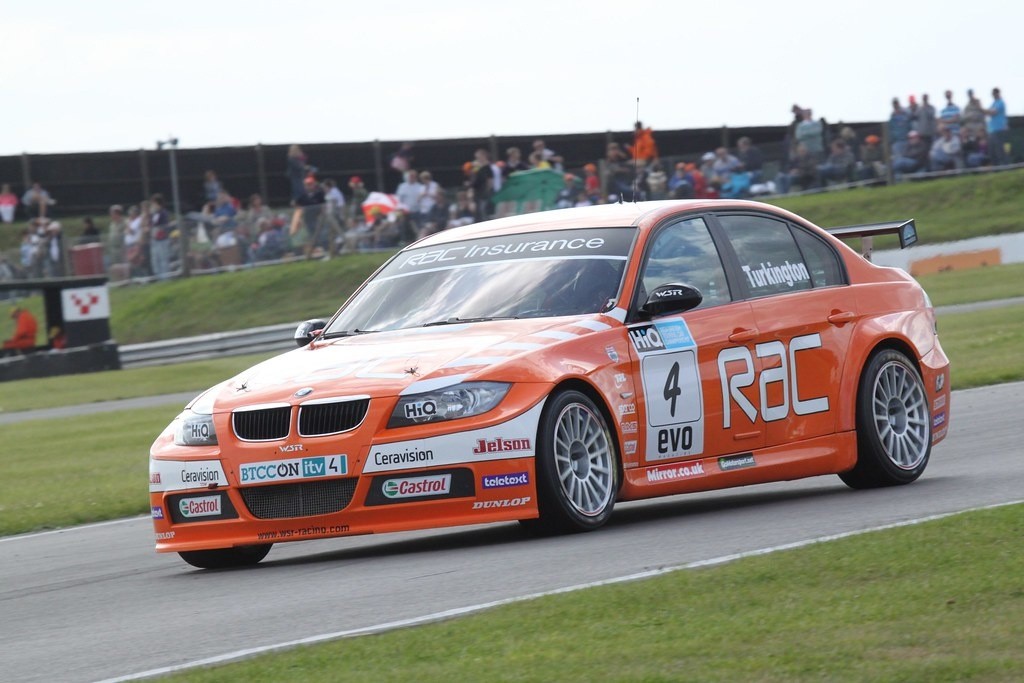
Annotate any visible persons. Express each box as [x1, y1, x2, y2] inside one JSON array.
[[47, 325, 66, 348], [1, 302, 38, 348], [2, 86, 1023, 276]]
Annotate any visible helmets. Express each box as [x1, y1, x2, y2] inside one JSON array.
[[50, 326, 60, 338], [8, 306, 19, 315]]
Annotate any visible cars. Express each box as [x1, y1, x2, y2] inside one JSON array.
[[146, 192, 953, 567]]
